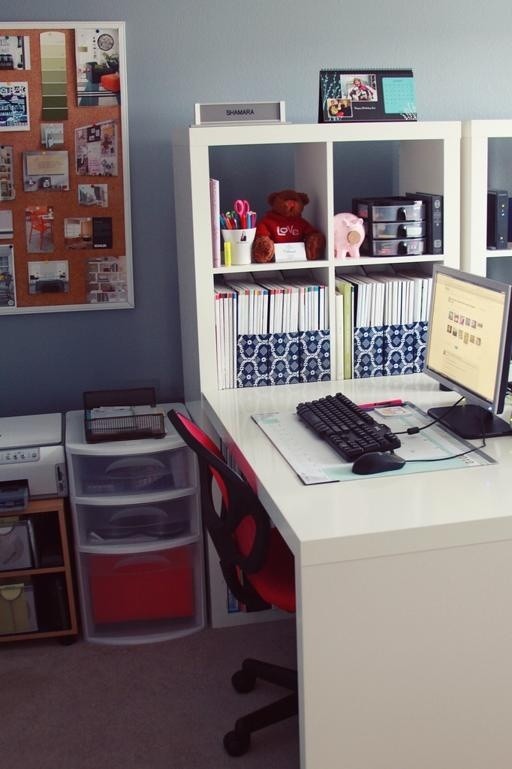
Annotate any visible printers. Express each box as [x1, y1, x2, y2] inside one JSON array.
[[0, 412, 69, 513]]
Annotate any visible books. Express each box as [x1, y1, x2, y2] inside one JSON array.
[[213, 272, 434, 390]]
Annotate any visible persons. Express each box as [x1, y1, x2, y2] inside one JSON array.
[[349, 77, 373, 100]]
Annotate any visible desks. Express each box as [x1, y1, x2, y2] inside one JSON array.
[[202, 371, 511, 767]]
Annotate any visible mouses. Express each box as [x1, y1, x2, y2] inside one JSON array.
[[353, 452, 405, 475]]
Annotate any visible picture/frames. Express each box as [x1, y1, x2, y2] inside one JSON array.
[[0, 20, 136, 317]]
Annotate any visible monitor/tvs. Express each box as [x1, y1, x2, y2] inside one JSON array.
[[422, 263, 512, 439]]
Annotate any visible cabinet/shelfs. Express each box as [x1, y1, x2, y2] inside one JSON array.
[[462, 117, 512, 287], [1, 498, 75, 648], [177, 122, 467, 409], [65, 409, 211, 644]]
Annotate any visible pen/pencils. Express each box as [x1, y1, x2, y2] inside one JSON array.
[[358, 400, 402, 408], [220, 211, 257, 229]]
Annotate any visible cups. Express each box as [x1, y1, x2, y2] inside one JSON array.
[[220, 227, 256, 266]]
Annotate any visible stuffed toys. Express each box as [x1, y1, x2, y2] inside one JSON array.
[[253, 189, 325, 263]]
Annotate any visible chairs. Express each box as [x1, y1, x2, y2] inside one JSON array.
[[166, 407, 298, 755]]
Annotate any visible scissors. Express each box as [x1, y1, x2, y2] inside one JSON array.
[[234, 200, 249, 219]]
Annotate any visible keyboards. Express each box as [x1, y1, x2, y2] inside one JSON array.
[[297, 392, 401, 462]]
